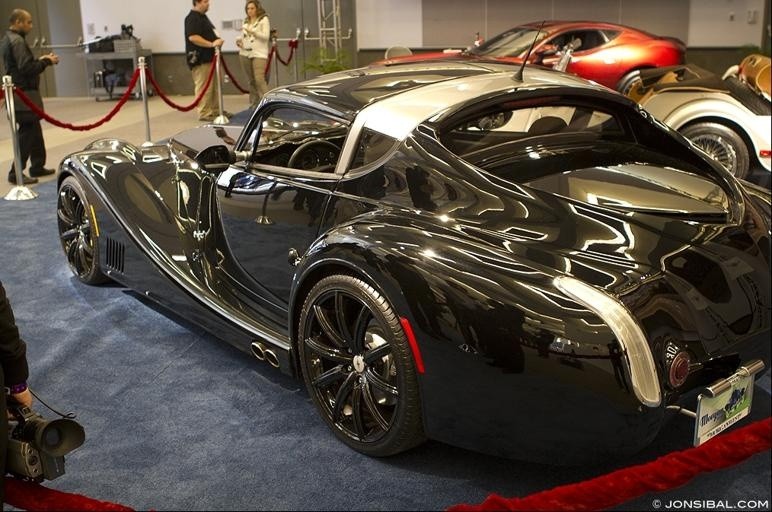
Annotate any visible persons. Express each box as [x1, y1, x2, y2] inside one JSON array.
[[237, 0, 270, 108], [1, 279, 33, 512], [184, 0, 233, 121], [0, 9, 59, 185]]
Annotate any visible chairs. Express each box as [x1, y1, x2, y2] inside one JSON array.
[[362, 137, 395, 164], [529, 115, 566, 136]]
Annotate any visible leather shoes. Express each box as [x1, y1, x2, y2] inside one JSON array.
[[30, 169, 55, 177], [9, 174, 37, 184]]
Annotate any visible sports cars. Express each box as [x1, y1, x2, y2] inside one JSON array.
[[637, 53, 772, 180], [372, 21, 686, 105], [57, 56, 772, 459]]
